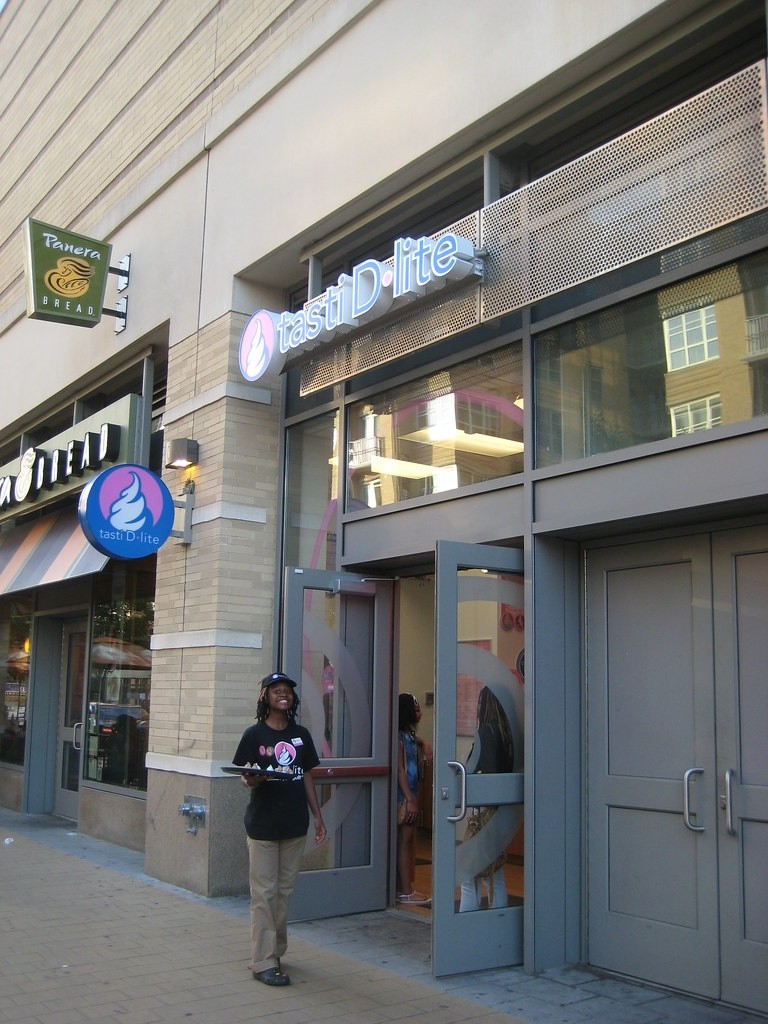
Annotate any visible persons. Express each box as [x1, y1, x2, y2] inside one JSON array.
[[232, 673, 327, 986], [456, 684, 524, 911], [397, 693, 433, 903]]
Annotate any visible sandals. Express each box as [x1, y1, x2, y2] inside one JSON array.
[[253, 967, 290, 986], [399, 890, 432, 904]]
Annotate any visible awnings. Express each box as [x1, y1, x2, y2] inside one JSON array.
[[1, 501, 109, 596]]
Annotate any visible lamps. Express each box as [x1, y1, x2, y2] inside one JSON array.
[[165, 438, 199, 470]]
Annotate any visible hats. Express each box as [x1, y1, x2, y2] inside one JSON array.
[[261, 672, 297, 688]]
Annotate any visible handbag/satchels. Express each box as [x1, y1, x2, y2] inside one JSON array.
[[463, 807, 506, 877], [397, 799, 408, 825]]
